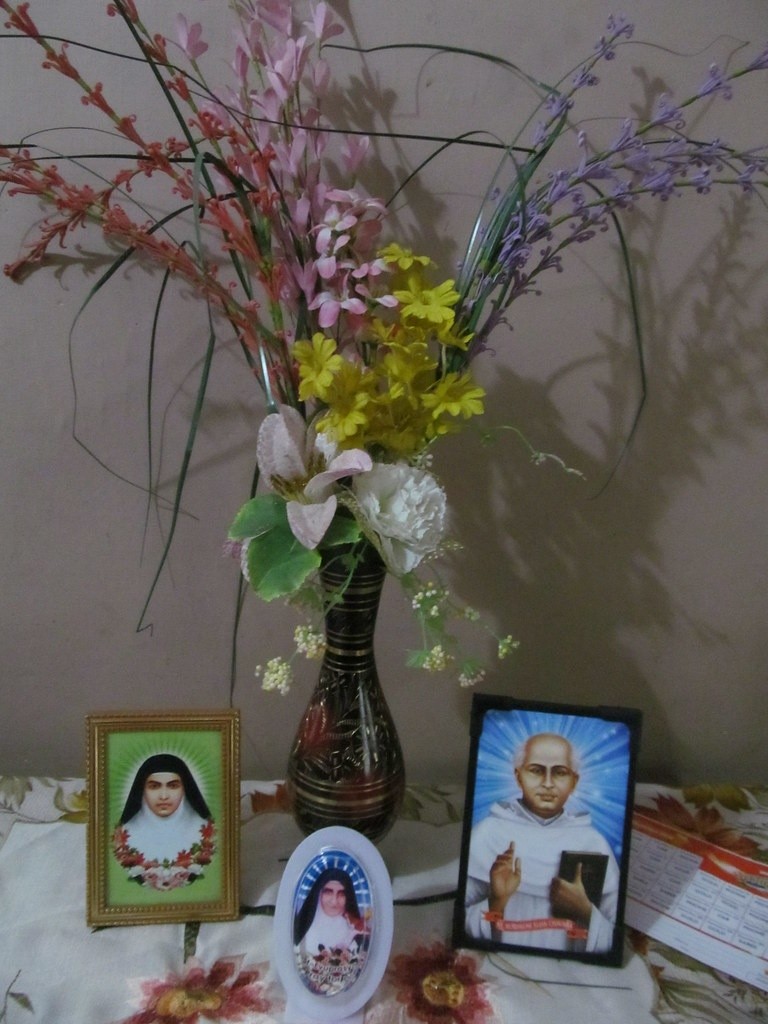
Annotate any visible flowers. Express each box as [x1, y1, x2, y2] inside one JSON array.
[[1, 0, 768, 713]]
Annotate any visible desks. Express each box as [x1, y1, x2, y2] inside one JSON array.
[[0, 777, 768, 1024]]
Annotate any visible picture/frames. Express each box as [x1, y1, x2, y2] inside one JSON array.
[[451, 692, 645, 969], [84, 708, 241, 928]]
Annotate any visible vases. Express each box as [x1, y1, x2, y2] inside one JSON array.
[[288, 532, 406, 845]]
